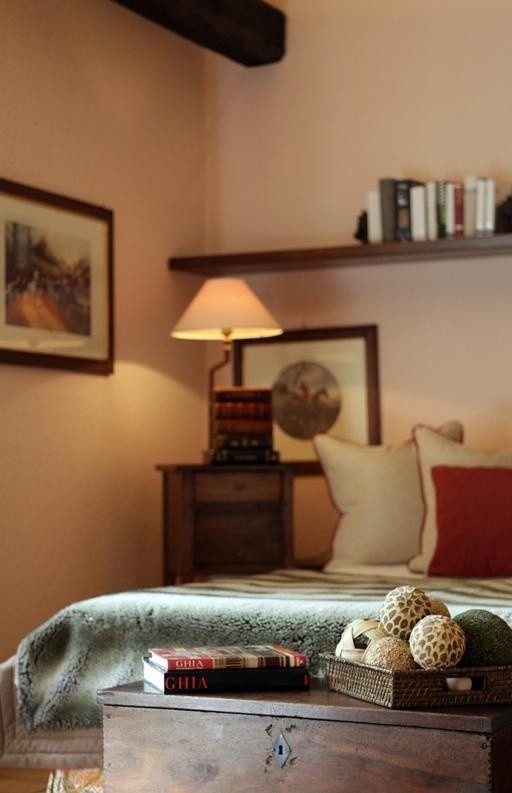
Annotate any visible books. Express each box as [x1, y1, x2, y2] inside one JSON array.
[[141, 655, 311, 695], [148, 642, 308, 671], [366, 173, 496, 244]]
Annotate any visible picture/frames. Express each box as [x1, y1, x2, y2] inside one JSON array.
[[0, 174, 117, 377], [225, 318, 383, 476]]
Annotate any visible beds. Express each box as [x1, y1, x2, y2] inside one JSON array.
[[64, 548, 512, 793]]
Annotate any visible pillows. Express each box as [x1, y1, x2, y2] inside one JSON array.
[[310, 413, 511, 578]]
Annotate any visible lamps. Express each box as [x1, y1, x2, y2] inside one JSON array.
[[166, 274, 282, 457]]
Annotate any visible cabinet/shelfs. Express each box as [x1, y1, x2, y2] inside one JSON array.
[[97, 681, 511, 793]]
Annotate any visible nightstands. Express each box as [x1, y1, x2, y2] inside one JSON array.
[[155, 457, 297, 587]]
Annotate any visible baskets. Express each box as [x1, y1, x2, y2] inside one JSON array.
[[318, 651, 512, 711]]
